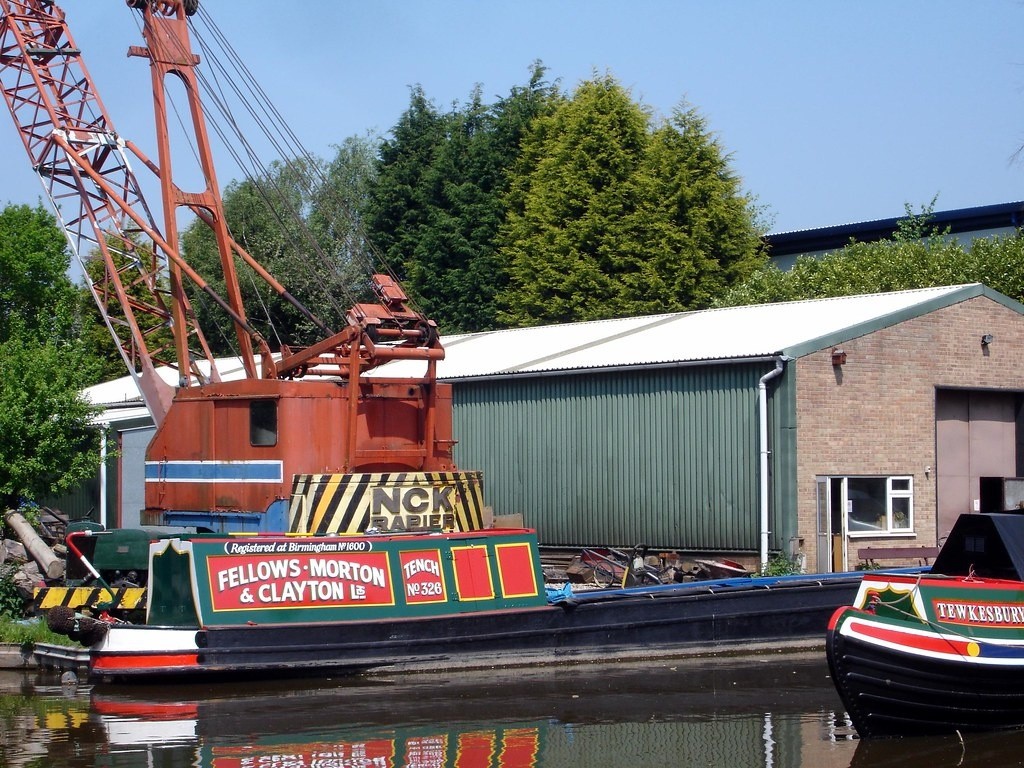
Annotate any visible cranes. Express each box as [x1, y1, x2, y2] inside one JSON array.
[[2, 3, 498, 531]]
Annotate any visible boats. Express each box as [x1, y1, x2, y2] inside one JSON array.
[[65, 527, 934, 687], [827, 512, 1024, 746]]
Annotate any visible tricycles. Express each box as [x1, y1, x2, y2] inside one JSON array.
[[580, 544, 667, 586]]
